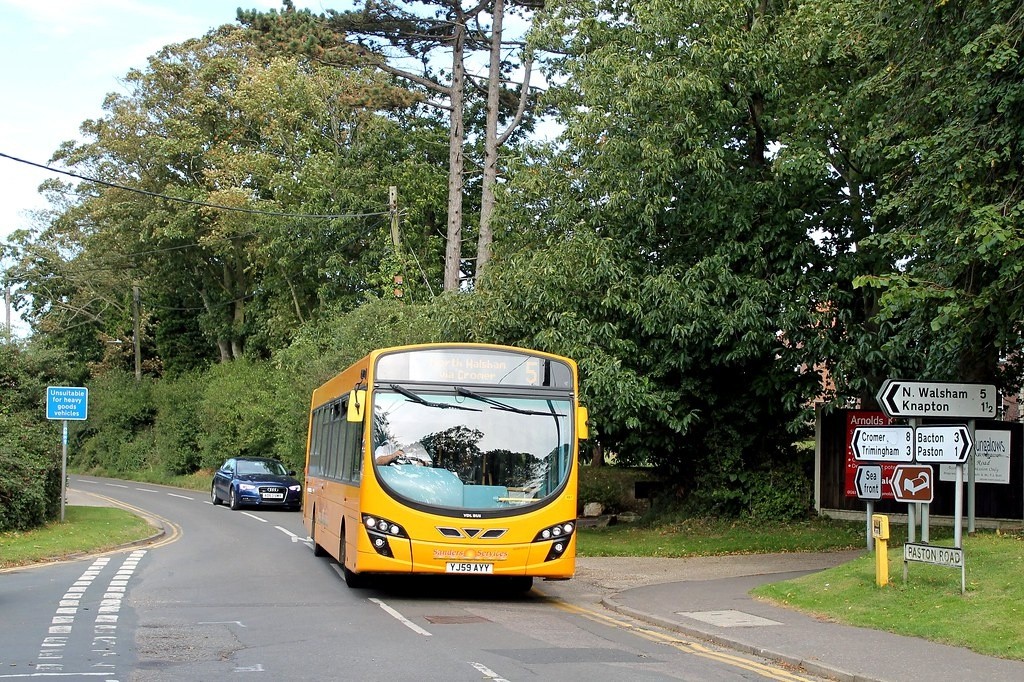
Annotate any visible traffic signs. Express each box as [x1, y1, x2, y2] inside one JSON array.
[[852, 465, 882, 500], [875, 377, 997, 422], [851, 424, 973, 463]]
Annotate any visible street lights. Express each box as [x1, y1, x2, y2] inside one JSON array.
[[106, 338, 142, 380]]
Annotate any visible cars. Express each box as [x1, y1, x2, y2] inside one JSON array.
[[210, 455, 303, 512]]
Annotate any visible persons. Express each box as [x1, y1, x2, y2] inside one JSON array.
[[375, 437, 434, 467]]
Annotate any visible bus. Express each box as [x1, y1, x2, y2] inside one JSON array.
[[298, 343, 592, 589]]
